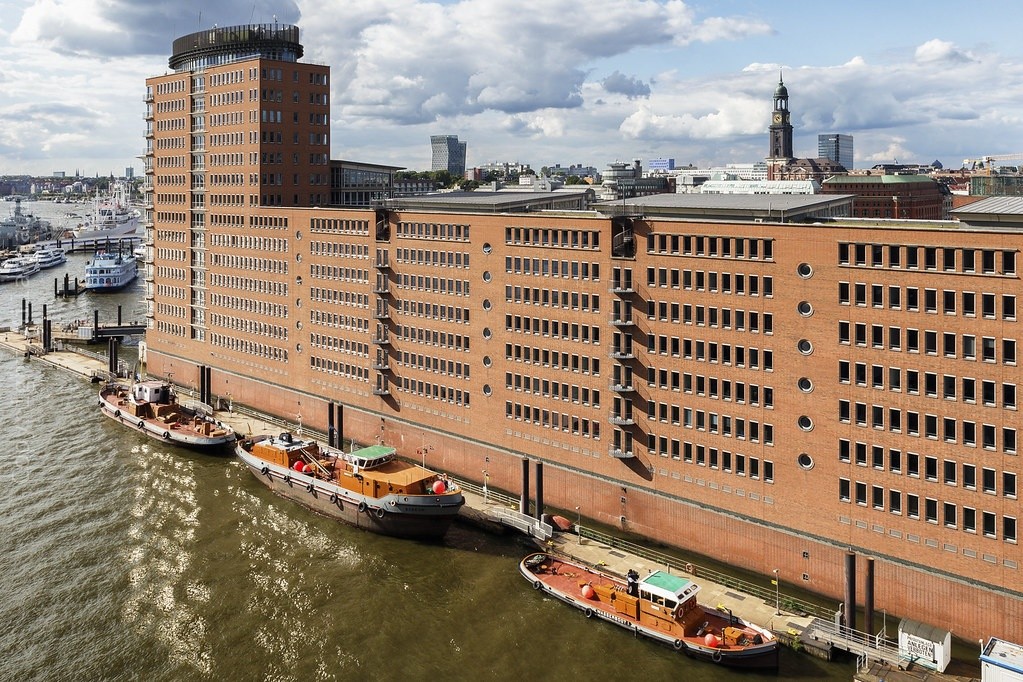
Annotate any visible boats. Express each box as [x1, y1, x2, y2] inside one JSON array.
[[0, 255, 40, 283], [79, 180, 141, 238], [234, 401, 465, 541], [84, 235, 139, 293], [33, 246, 67, 268], [97, 345, 235, 447], [0, 192, 93, 219], [852, 634, 1023, 682], [518, 553, 779, 665], [0, 196, 53, 251]]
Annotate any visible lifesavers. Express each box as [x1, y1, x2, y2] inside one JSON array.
[[678, 608, 684, 618], [690, 600, 695, 609]]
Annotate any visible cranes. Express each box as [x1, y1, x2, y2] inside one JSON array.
[[963, 154, 1023, 198]]
